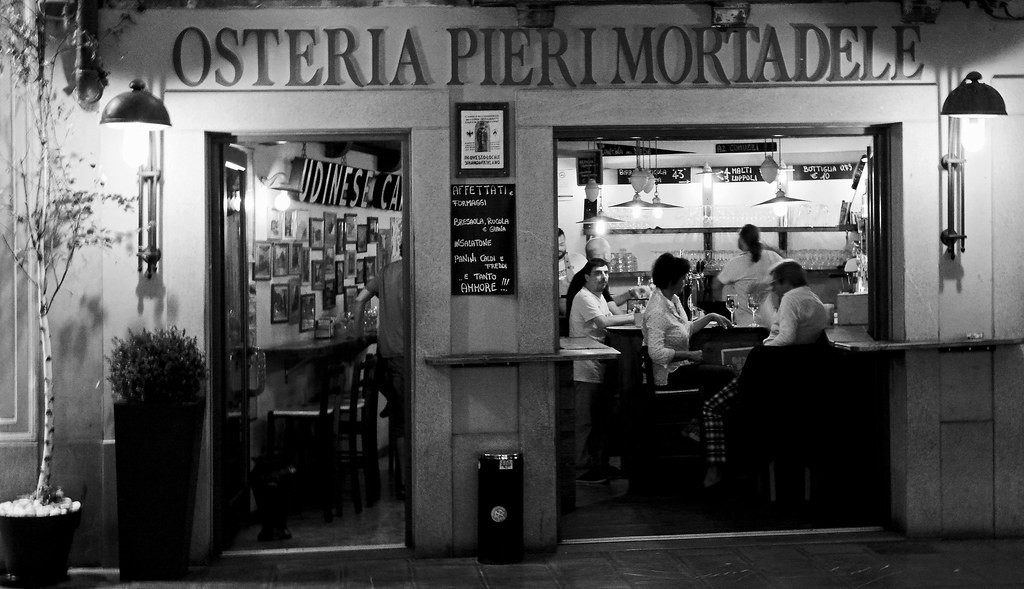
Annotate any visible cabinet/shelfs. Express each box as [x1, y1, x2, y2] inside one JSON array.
[[582, 225, 859, 278]]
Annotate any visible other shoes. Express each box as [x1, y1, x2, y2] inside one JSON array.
[[702, 466, 722, 487], [572, 463, 607, 485], [680, 424, 701, 442]]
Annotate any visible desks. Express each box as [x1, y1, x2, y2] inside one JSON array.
[[263, 331, 378, 385]]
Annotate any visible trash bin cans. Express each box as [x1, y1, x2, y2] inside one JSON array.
[[476, 448, 525, 564]]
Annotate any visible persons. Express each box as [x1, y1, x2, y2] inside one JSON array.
[[703, 262, 829, 489], [640, 253, 737, 440], [556, 228, 644, 482], [707, 222, 784, 327], [349, 243, 406, 430]]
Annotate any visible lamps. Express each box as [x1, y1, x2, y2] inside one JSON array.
[[585, 141, 600, 202], [98, 78, 172, 134], [642, 140, 656, 193], [608, 140, 654, 219], [260, 172, 305, 213], [751, 138, 812, 218], [575, 137, 625, 236], [940, 72, 1009, 153], [642, 136, 685, 220], [516, 3, 556, 29], [759, 139, 780, 184], [900, 0, 943, 25], [710, 0, 752, 29], [630, 138, 648, 193]]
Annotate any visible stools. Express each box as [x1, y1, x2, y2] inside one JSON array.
[[736, 343, 852, 503], [635, 342, 709, 511], [264, 352, 407, 524]]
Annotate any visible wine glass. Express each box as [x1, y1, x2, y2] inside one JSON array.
[[746, 294, 761, 327], [787, 249, 849, 270], [652, 249, 742, 272], [725, 294, 740, 328]]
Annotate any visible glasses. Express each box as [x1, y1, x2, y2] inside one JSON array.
[[770, 277, 783, 286]]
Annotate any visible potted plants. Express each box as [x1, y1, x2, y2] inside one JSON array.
[[0, 0, 139, 589], [110, 328, 212, 584]]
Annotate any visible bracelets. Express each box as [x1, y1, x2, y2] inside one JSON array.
[[629, 290, 634, 298]]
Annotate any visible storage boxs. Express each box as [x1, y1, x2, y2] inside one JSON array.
[[837, 293, 868, 325]]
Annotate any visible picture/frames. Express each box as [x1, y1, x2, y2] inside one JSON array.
[[299, 293, 316, 333], [266, 207, 379, 255], [344, 285, 359, 320], [252, 240, 272, 281], [344, 249, 357, 280], [311, 259, 325, 290], [335, 260, 345, 295], [363, 256, 376, 286], [322, 279, 337, 310], [300, 246, 311, 286], [289, 242, 301, 276], [354, 259, 364, 285], [376, 229, 391, 272], [358, 288, 371, 310], [270, 283, 290, 324], [272, 242, 290, 277], [454, 102, 511, 179], [323, 245, 335, 274]]
